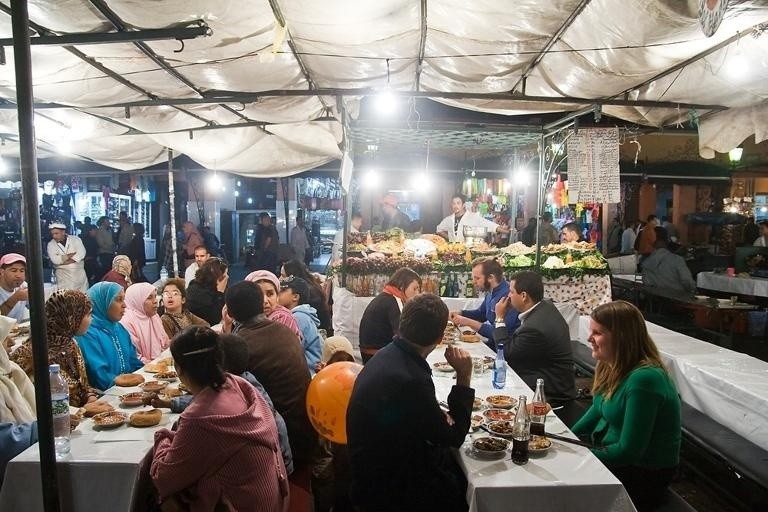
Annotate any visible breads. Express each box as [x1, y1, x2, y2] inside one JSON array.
[[129, 409, 161, 426], [144, 362, 170, 373], [462, 335, 481, 343], [528, 403, 552, 415], [115, 374, 145, 387], [83, 400, 114, 417]]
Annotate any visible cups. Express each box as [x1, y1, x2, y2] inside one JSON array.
[[471, 356, 484, 378]]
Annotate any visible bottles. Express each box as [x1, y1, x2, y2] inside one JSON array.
[[530, 378, 546, 437], [446, 273, 453, 297], [354, 270, 440, 297], [453, 272, 459, 297], [48, 364, 71, 457], [491, 344, 506, 390], [511, 395, 529, 465], [439, 272, 446, 297], [466, 267, 474, 298]]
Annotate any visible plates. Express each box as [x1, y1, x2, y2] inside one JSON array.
[[138, 380, 170, 392], [471, 437, 512, 461], [485, 420, 513, 438], [116, 391, 149, 407], [93, 410, 130, 430], [433, 361, 456, 372], [153, 370, 178, 383], [472, 393, 484, 413], [485, 393, 518, 410], [526, 434, 553, 459], [482, 407, 514, 421]]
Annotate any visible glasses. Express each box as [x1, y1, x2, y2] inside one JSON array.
[[161, 290, 182, 297]]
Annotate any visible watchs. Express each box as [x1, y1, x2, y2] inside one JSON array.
[[495, 318, 505, 323]]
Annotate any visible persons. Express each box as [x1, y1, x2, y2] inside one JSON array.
[[46, 219, 91, 293], [114, 211, 133, 256], [183, 247, 210, 291], [343, 292, 475, 512], [289, 217, 311, 264], [445, 258, 520, 352], [120, 283, 171, 366], [533, 211, 560, 245], [493, 272, 577, 409], [95, 214, 115, 276], [73, 280, 144, 390], [241, 270, 299, 346], [248, 214, 273, 270], [304, 227, 318, 267], [10, 290, 97, 414], [571, 297, 682, 512], [73, 218, 84, 238], [124, 223, 150, 283], [606, 218, 623, 253], [150, 327, 292, 512], [0, 312, 83, 477], [743, 217, 761, 246], [269, 276, 320, 386], [642, 238, 698, 297], [158, 279, 212, 341], [637, 214, 658, 255], [371, 216, 383, 232], [257, 213, 280, 269], [434, 193, 511, 245], [376, 195, 413, 236], [661, 215, 680, 242], [160, 223, 177, 276], [201, 227, 220, 257], [509, 216, 524, 244], [0, 253, 30, 325], [559, 223, 588, 245], [214, 333, 292, 482], [81, 215, 91, 243], [85, 226, 97, 280], [353, 266, 424, 368], [182, 256, 230, 326], [522, 217, 537, 245], [619, 222, 636, 254], [331, 210, 363, 268], [277, 259, 331, 339], [219, 280, 317, 459], [179, 221, 204, 270], [752, 220, 768, 247], [102, 254, 133, 292]]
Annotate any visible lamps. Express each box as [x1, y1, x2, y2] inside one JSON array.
[[728, 144, 743, 172]]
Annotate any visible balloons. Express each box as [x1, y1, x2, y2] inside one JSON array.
[[306, 361, 365, 445]]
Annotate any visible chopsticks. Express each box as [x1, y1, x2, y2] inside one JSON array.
[[544, 431, 593, 449]]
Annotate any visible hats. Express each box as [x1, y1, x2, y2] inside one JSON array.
[[1, 251, 28, 269], [375, 194, 401, 208], [243, 269, 281, 292], [280, 277, 309, 305]]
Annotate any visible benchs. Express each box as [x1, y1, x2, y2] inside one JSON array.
[[554, 400, 696, 512], [570, 340, 768, 491]]
